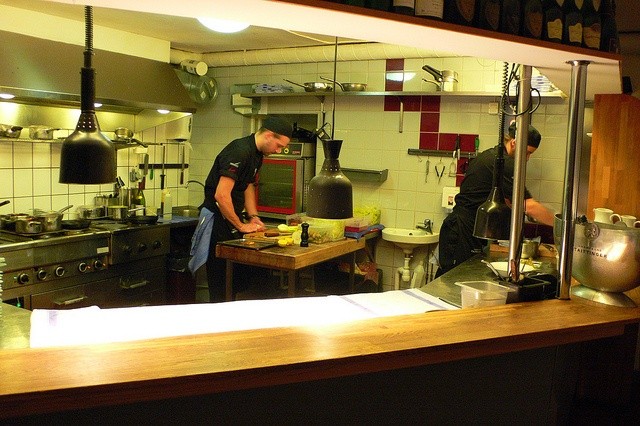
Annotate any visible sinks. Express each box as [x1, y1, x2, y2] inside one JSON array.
[[382, 228, 439, 244]]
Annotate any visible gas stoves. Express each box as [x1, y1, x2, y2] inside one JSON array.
[[0, 220, 171, 300]]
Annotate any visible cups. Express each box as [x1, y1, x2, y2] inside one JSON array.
[[183, 210, 190, 217], [108, 197, 120, 206]]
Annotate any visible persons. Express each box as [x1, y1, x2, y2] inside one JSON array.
[[188, 118, 293, 302], [434, 122, 554, 278]]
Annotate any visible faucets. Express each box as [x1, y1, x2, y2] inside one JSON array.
[[415, 219, 434, 234]]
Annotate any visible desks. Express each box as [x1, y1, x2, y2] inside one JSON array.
[[215, 229, 366, 300]]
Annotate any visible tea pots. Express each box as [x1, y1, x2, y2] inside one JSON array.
[[593, 208, 621, 225], [621, 215, 640, 229]]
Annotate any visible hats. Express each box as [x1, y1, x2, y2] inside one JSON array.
[[261, 117, 294, 138], [508, 122, 541, 149]]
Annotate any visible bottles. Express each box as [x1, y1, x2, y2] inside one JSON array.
[[128, 187, 131, 216], [444, 0, 478, 29], [135, 182, 146, 216], [124, 186, 127, 206], [163, 192, 172, 220], [500, 0, 521, 36], [581, 1, 601, 51], [478, 0, 500, 31], [136, 186, 139, 195], [597, 0, 617, 52], [545, 0, 565, 43], [119, 187, 124, 206], [562, 0, 583, 47], [389, 0, 415, 17], [521, 0, 544, 40], [161, 193, 166, 217], [300, 223, 309, 247], [415, 0, 444, 21], [131, 186, 135, 216]]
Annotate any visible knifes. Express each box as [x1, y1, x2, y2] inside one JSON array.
[[265, 233, 292, 237], [457, 137, 461, 161], [180, 146, 184, 184], [142, 154, 149, 189], [438, 166, 445, 184], [542, 245, 554, 252], [453, 140, 458, 160]]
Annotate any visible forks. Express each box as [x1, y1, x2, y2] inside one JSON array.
[[160, 146, 166, 189]]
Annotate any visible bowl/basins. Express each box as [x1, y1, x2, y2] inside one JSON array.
[[7, 214, 29, 221], [308, 224, 335, 244], [77, 205, 105, 219], [521, 240, 539, 259], [553, 213, 640, 308]]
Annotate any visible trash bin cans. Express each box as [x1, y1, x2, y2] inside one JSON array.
[[167, 252, 196, 304]]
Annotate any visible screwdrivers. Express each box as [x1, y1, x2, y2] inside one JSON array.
[[475, 137, 479, 157]]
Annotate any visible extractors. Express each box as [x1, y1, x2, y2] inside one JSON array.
[[0, 30, 197, 133]]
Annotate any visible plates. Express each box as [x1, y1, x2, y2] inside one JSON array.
[[487, 262, 534, 278]]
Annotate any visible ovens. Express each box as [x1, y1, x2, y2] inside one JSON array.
[[253, 142, 314, 219]]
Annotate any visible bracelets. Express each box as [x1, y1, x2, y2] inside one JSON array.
[[246, 215, 259, 219]]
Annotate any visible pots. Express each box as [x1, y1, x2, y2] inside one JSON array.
[[36, 205, 73, 231], [0, 215, 10, 229], [29, 125, 60, 140], [16, 217, 43, 234], [320, 76, 368, 91], [283, 79, 334, 92], [422, 78, 457, 92], [94, 194, 108, 206], [114, 127, 148, 148], [422, 65, 458, 82], [127, 213, 172, 224], [61, 215, 113, 230], [107, 206, 146, 219], [0, 124, 23, 138]]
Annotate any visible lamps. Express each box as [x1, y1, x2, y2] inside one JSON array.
[[473, 64, 520, 239], [307, 36, 352, 218], [59, 6, 117, 184]]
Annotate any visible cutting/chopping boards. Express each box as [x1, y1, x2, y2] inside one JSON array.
[[243, 227, 292, 240], [490, 243, 556, 257]]
